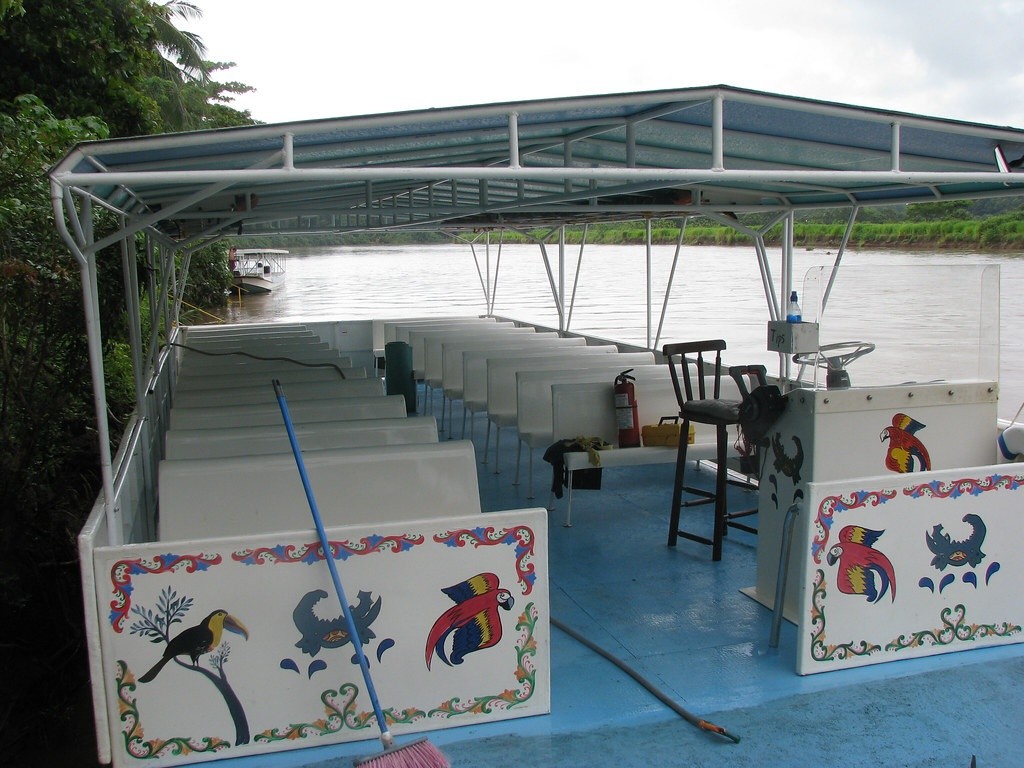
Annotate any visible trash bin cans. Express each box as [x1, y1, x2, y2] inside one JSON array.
[[383, 342, 417, 416]]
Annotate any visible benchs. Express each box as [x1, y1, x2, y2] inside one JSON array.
[[157, 314, 753, 541]]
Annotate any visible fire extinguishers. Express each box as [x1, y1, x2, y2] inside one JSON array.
[[612, 368, 641, 449]]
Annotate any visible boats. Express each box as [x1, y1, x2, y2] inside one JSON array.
[[226, 247, 290, 293], [47, 74, 1023, 767]]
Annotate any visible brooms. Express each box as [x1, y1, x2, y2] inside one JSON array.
[[270, 374, 454, 768]]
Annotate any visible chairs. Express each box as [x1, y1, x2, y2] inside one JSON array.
[[662, 339, 762, 563]]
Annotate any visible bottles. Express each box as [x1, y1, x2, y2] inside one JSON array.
[[786, 290, 801, 324]]
